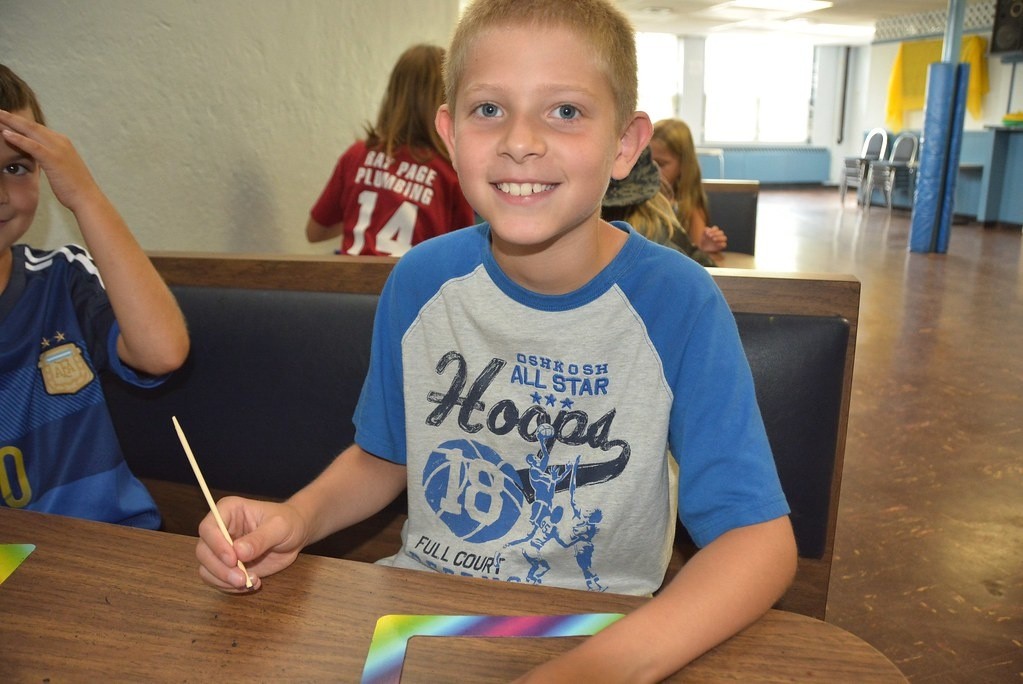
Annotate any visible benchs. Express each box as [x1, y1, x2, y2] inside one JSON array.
[[106, 254, 861, 619]]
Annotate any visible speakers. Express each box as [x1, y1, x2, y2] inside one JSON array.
[[990, 0, 1023, 54]]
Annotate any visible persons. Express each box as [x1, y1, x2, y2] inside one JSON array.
[[0, 66, 188, 530], [194, 1, 798, 684]]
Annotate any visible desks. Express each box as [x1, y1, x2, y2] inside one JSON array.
[[0, 502, 912, 684], [696, 149, 725, 180]]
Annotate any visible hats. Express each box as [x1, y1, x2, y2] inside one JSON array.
[[601, 144, 661, 206]]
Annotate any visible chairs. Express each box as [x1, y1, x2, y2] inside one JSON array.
[[840, 129, 918, 213]]
